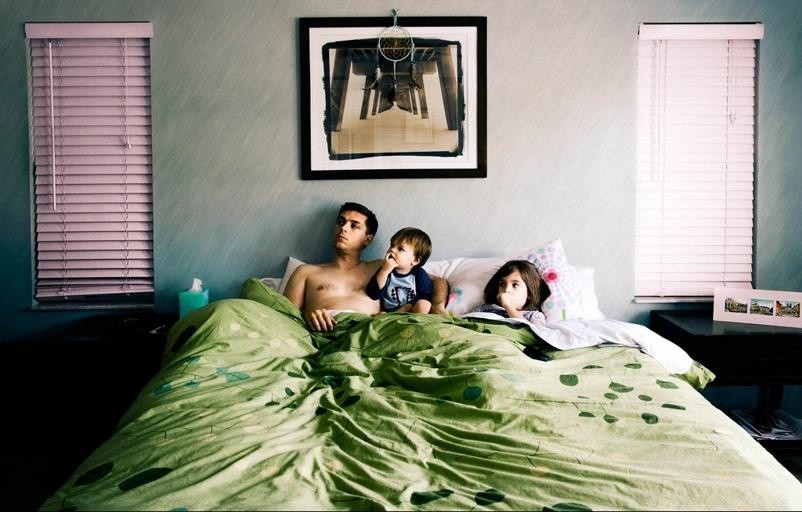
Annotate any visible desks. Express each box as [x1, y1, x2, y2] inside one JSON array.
[[650, 312, 801, 464]]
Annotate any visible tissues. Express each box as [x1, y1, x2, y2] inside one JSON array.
[[179, 277, 209, 317]]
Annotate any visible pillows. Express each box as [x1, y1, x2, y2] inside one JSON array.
[[506, 238, 580, 316], [278, 255, 309, 295], [548, 267, 607, 321]]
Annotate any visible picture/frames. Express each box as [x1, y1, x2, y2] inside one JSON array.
[[294, 15, 489, 180], [713, 287, 801, 329]]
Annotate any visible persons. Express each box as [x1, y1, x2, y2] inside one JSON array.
[[469, 258, 548, 329], [366, 226, 434, 317], [279, 202, 450, 334]]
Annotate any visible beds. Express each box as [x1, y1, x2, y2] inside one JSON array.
[[40, 238, 801, 511]]
